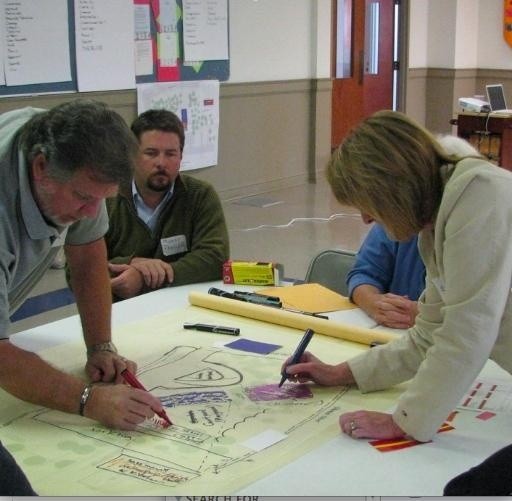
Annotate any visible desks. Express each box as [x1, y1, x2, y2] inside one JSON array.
[[0, 271, 512, 499], [447, 107, 512, 174]]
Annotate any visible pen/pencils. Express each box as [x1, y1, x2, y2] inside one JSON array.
[[232, 290, 281, 307], [120, 369, 172, 425], [278, 328, 315, 388], [184, 324, 241, 336]]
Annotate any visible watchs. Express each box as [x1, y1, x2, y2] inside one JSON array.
[[86, 341, 118, 354]]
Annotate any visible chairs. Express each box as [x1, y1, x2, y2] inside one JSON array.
[[301, 248, 365, 302]]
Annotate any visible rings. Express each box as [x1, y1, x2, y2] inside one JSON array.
[[350, 421, 355, 430]]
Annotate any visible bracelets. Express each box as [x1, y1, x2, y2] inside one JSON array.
[[78, 383, 93, 417]]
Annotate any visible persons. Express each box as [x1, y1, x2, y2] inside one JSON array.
[[280, 111, 512, 443], [63, 108, 229, 302], [346, 133, 484, 329], [0, 99, 162, 431]]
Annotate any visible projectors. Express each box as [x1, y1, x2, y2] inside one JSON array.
[[458, 97, 491, 113]]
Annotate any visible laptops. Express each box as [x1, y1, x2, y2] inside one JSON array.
[[485, 83, 512, 115]]
